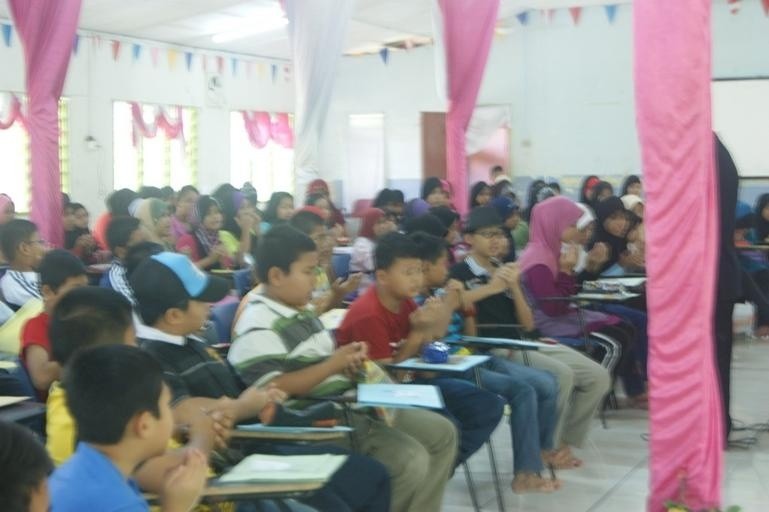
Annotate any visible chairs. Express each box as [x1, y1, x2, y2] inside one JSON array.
[[0, 271, 649, 510]]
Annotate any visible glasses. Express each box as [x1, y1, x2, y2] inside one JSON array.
[[473, 230, 503, 239]]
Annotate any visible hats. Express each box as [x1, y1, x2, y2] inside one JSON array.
[[597, 196, 624, 222], [487, 196, 519, 220], [131, 251, 229, 302]]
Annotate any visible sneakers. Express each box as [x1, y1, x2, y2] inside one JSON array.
[[512, 476, 558, 493], [541, 447, 582, 468]]
